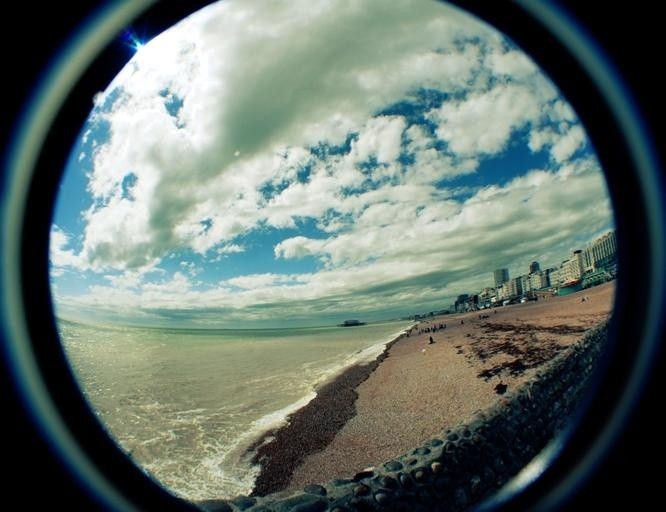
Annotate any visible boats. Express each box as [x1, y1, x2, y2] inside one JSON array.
[[337, 319, 367, 327]]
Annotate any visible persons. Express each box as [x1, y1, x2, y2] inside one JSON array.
[[405, 323, 445, 337], [429, 336, 433, 344]]
